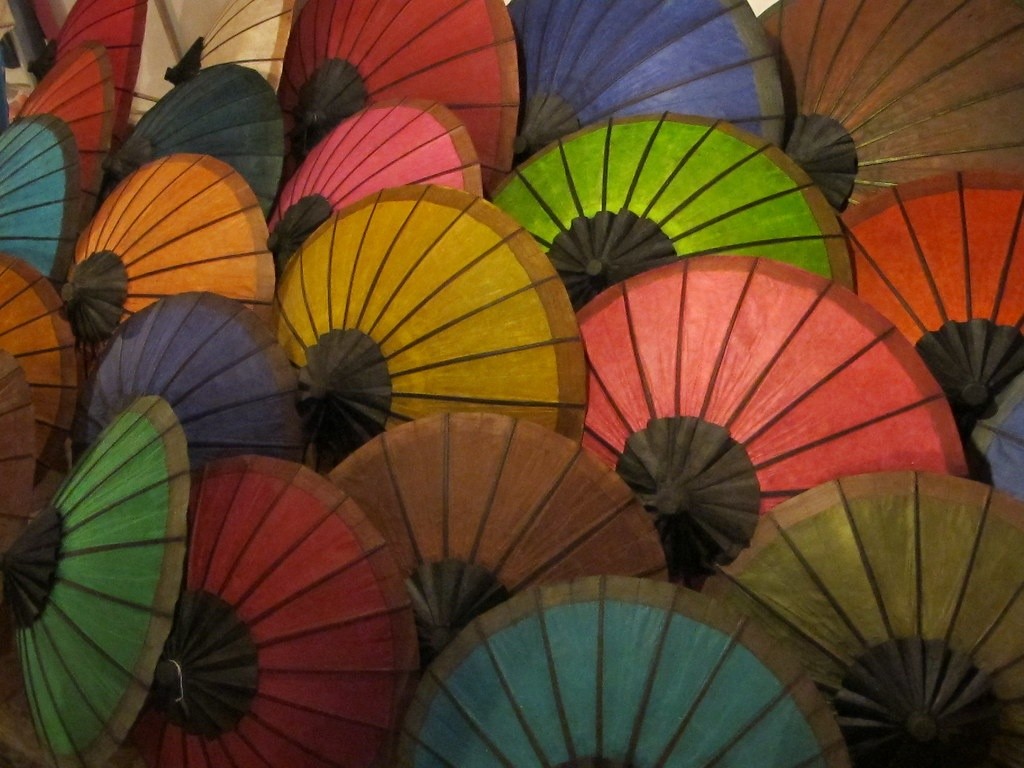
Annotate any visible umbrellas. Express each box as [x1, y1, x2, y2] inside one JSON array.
[[0, 0, 1024, 661], [700, 469, 1024, 768], [0, 393, 189, 768], [402, 576, 844, 768], [125, 456, 420, 768]]
